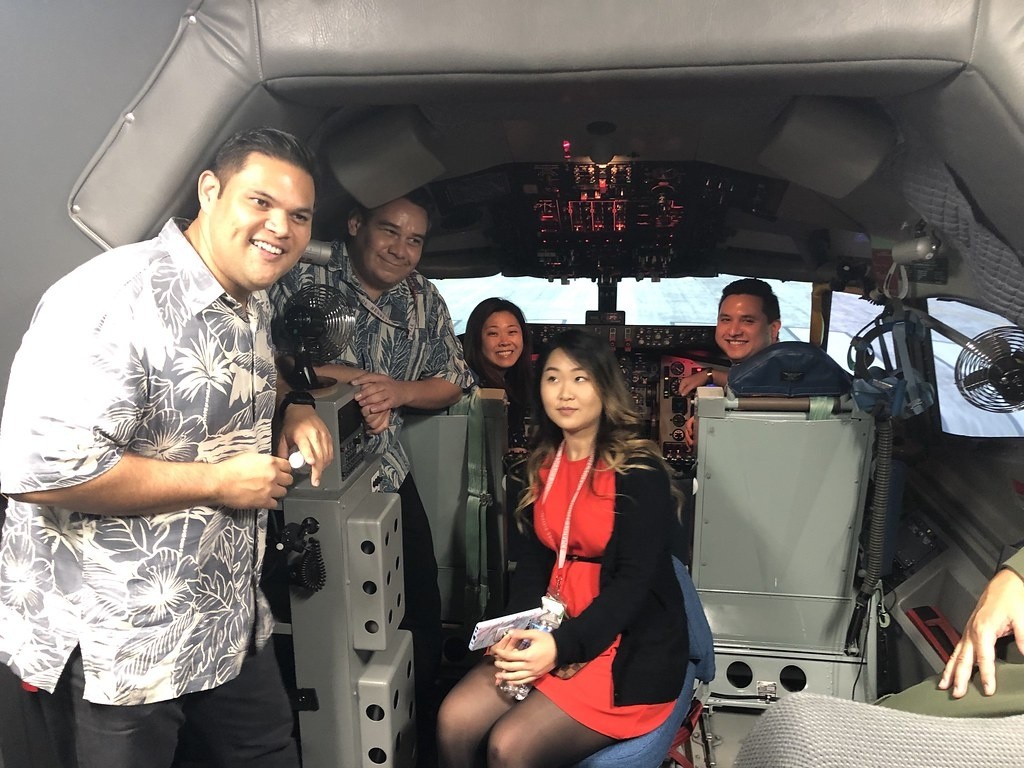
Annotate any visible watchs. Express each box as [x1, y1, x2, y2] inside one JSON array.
[[279, 392, 315, 420]]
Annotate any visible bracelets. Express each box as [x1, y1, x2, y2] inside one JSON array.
[[707, 367, 713, 384]]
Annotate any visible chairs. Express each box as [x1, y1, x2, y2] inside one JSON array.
[[397, 388, 517, 625], [686, 391, 872, 703], [579, 553, 717, 768]]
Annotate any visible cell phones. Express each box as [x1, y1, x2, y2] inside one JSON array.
[[469, 607, 543, 652]]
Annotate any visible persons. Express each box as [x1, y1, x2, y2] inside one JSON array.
[[265, 180, 472, 768], [0, 130, 333, 768], [437, 329, 690, 768], [670, 279, 782, 546], [464, 297, 532, 471], [870, 547, 1023, 718]]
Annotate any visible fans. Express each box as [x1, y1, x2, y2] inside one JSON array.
[[905, 307, 1024, 414], [274, 283, 355, 392]]
